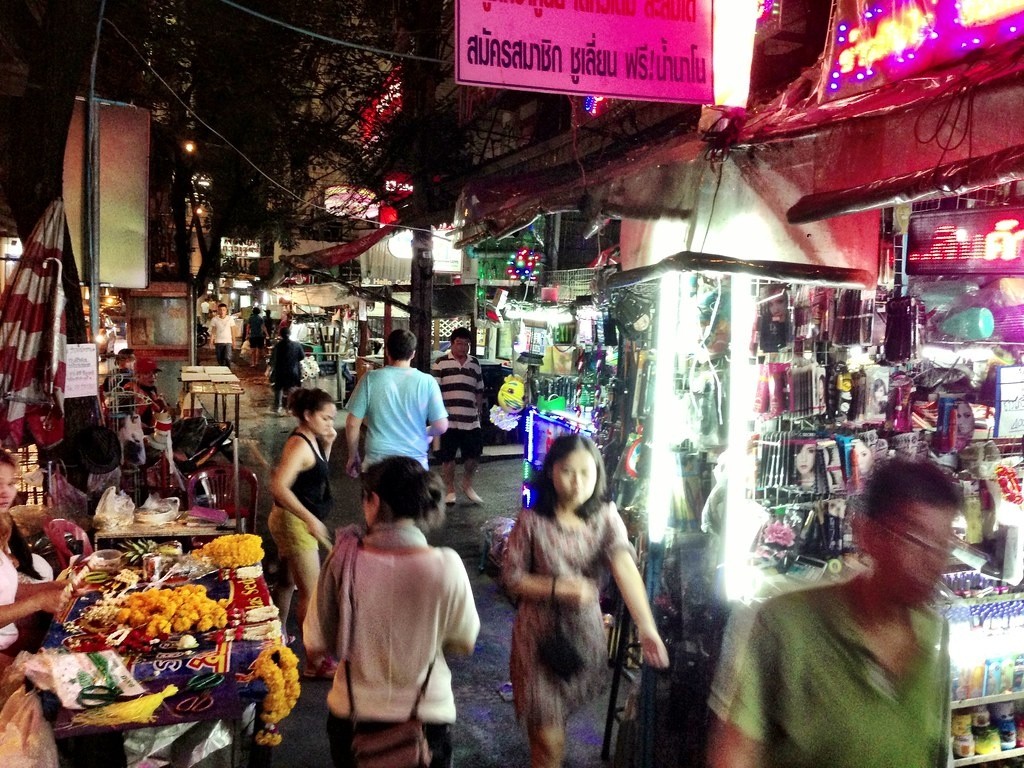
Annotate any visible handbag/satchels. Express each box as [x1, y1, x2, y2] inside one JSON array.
[[352, 718, 431, 768], [134, 493, 180, 523], [264, 366, 274, 377], [92, 485, 134, 530], [536, 577, 608, 677]]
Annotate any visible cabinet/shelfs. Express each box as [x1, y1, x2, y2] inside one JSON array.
[[846, 554, 1024, 768]]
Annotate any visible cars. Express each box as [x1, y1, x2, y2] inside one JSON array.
[[78, 283, 126, 336]]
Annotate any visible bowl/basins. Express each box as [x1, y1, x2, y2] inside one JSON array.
[[90, 549, 122, 574]]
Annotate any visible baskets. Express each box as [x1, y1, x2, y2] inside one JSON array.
[[543, 268, 598, 296]]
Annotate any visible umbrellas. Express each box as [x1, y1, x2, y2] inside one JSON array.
[[0, 194, 67, 450]]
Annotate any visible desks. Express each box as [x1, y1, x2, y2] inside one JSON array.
[[179, 370, 245, 440], [37, 563, 286, 768], [93, 511, 245, 553]]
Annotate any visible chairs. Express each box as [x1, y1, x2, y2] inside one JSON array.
[[187, 465, 259, 548], [39, 514, 93, 569]]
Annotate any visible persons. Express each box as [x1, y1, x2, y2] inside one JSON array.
[[209, 299, 217, 321], [428, 328, 484, 504], [264, 327, 305, 415], [767, 286, 787, 325], [874, 381, 889, 417], [796, 444, 817, 490], [500, 436, 670, 768], [200, 299, 210, 324], [708, 464, 957, 768], [303, 456, 481, 768], [209, 304, 236, 370], [345, 329, 448, 487], [246, 308, 269, 367], [104, 348, 157, 407], [957, 404, 975, 438], [268, 389, 339, 678], [0, 450, 71, 654], [854, 440, 875, 482]]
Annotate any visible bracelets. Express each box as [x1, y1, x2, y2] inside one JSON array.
[[551, 577, 557, 603]]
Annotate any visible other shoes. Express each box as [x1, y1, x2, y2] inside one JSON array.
[[445, 493, 456, 507], [458, 482, 482, 503]]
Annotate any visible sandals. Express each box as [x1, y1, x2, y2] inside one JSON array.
[[304, 656, 338, 678]]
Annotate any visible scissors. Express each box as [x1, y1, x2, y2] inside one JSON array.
[[175, 689, 215, 713], [77, 685, 139, 708], [82, 571, 115, 584], [165, 672, 225, 700]]
